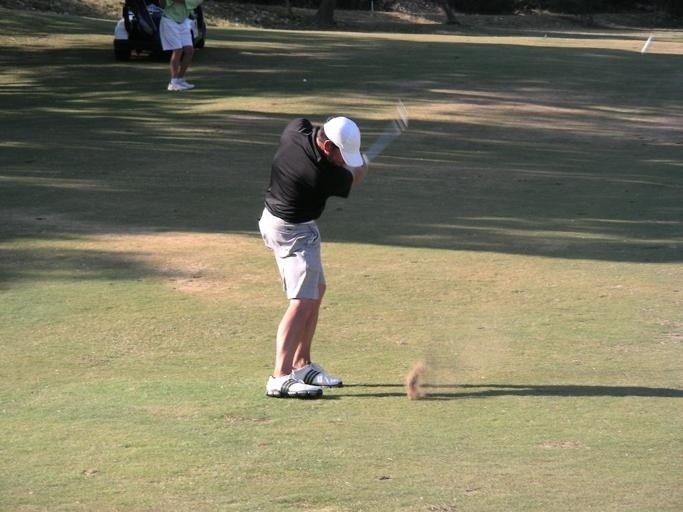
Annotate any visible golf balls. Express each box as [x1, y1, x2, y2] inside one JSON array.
[[303, 78, 306, 80]]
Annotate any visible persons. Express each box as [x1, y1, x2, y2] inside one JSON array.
[[159, 0, 196, 92], [257, 116, 368, 399]]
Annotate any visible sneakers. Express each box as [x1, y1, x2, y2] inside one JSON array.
[[167, 82, 195, 91], [291, 362, 342, 387], [266, 375, 323, 399]]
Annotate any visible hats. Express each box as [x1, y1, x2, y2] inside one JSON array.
[[323, 116, 364, 168]]
